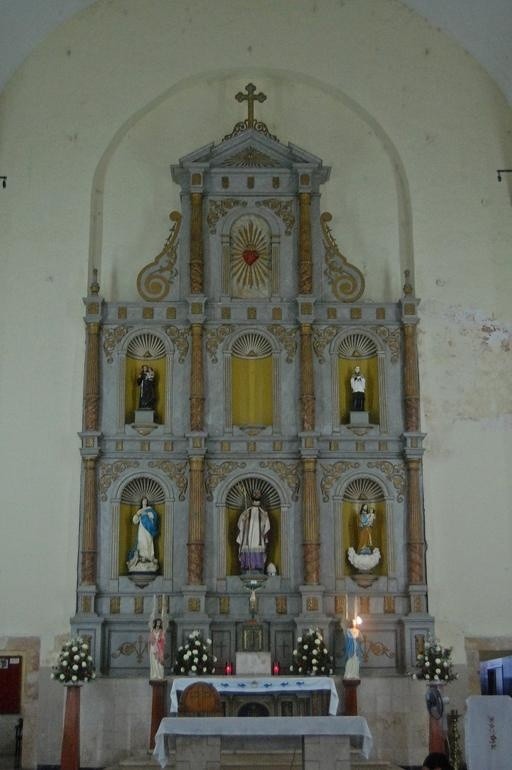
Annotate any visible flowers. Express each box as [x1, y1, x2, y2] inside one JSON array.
[[286, 626, 331, 677], [50, 633, 95, 684], [413, 631, 458, 684], [173, 628, 221, 676]]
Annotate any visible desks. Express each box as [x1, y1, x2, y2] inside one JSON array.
[[152, 715, 375, 770]]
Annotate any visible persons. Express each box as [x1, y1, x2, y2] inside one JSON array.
[[145, 366, 155, 382], [148, 618, 167, 680], [137, 364, 156, 409], [423, 752, 448, 770], [132, 496, 158, 561], [343, 620, 363, 678], [366, 508, 375, 525], [357, 503, 373, 553], [236, 489, 271, 570], [350, 365, 366, 411]]
